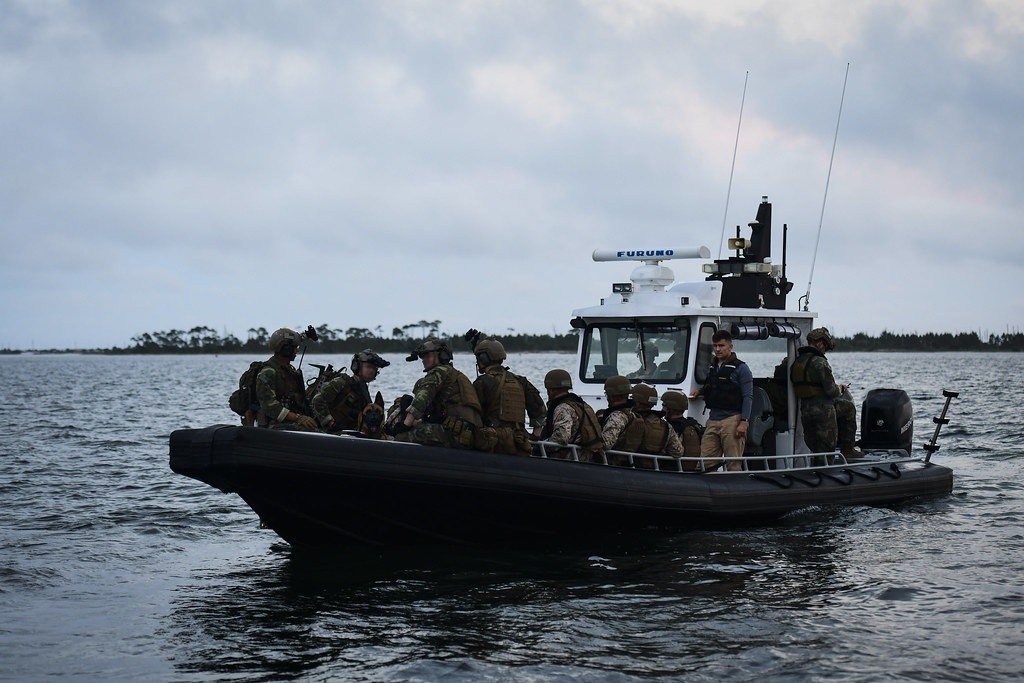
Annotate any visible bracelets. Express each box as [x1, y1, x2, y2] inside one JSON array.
[[740, 419, 748, 421]]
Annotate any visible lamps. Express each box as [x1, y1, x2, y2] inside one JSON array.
[[613, 282, 632, 292]]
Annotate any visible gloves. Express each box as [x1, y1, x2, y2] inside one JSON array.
[[325, 420, 342, 432], [393, 421, 414, 433], [295, 414, 317, 430], [528, 432, 541, 440]]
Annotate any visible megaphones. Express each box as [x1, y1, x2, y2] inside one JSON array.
[[727, 238, 752, 250]]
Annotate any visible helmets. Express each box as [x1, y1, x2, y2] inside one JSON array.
[[544, 369, 572, 390], [350, 349, 390, 380], [630, 383, 657, 405], [418, 338, 453, 362], [661, 392, 688, 410], [474, 338, 506, 360], [635, 342, 659, 357], [603, 376, 630, 395], [269, 328, 301, 351], [807, 327, 833, 351]]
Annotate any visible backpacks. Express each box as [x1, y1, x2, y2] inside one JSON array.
[[304, 364, 367, 418], [228, 361, 282, 418]]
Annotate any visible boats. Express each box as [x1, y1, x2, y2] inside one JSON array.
[[170, 62, 961, 571]]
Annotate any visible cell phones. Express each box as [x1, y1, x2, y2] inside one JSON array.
[[848, 383, 851, 386]]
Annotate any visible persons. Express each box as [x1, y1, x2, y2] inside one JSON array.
[[601, 375, 633, 454], [790, 327, 865, 467], [625, 340, 659, 379], [473, 338, 547, 457], [394, 339, 484, 450], [312, 349, 390, 434], [256, 328, 321, 433], [653, 335, 687, 377], [607, 384, 684, 470], [661, 391, 705, 471], [533, 369, 606, 462], [690, 330, 753, 472]]
